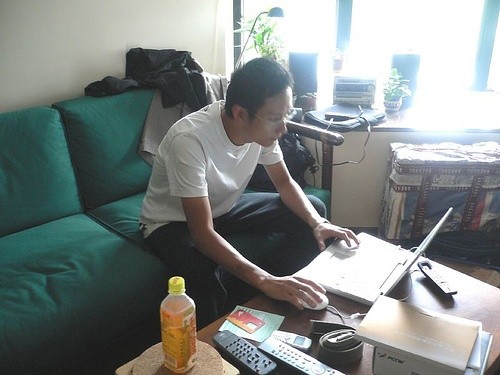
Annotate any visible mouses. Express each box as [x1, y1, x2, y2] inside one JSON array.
[[298, 288, 329, 311]]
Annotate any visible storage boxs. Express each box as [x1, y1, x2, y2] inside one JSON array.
[[371, 331, 493, 375]]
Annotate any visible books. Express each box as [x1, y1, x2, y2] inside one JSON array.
[[355, 295, 493, 375]]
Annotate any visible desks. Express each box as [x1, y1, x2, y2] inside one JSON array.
[[115, 231, 500, 375]]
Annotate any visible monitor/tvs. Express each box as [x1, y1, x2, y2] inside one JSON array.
[[292, 207, 454, 305]]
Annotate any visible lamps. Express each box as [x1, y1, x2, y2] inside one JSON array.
[[236, 8, 284, 68]]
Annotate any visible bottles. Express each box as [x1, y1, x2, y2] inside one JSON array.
[[160, 275, 198, 374]]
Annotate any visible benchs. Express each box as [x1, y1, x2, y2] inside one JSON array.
[[0, 71, 343, 375]]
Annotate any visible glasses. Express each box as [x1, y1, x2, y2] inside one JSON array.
[[249, 107, 296, 126]]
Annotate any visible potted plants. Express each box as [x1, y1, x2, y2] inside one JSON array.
[[382, 69, 410, 112]]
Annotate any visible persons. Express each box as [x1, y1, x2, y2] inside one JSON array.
[[140, 58, 361, 331]]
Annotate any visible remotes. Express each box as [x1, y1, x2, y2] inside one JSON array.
[[213, 330, 277, 375], [258, 337, 346, 375], [271, 330, 312, 352]]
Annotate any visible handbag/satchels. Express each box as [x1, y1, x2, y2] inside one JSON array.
[[303, 104, 386, 132], [125, 48, 208, 112]]
